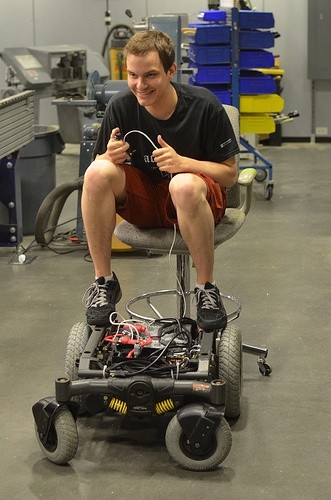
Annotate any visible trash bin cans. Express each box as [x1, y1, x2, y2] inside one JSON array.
[[19, 124, 65, 235]]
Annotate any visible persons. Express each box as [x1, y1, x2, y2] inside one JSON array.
[[80, 29, 240, 329]]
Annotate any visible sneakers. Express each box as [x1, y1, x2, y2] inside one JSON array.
[[191, 282, 227, 331], [82, 271, 122, 325]]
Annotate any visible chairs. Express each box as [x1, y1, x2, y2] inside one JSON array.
[[114, 103, 256, 331]]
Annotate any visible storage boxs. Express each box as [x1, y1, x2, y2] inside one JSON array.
[[185, 10, 284, 135]]
